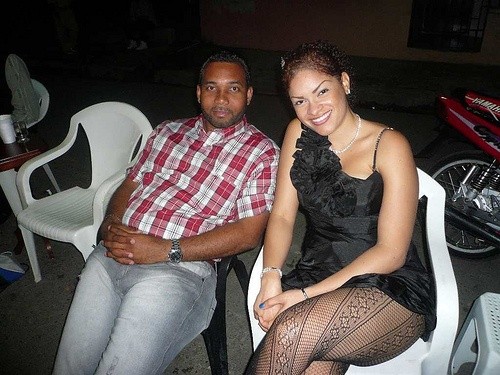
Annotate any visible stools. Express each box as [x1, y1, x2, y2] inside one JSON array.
[[448, 291, 500, 375]]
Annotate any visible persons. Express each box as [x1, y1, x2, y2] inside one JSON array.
[[52, 52, 280, 375], [241, 38, 443, 375]]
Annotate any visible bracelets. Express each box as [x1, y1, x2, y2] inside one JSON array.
[[260, 267, 284, 279], [299, 287, 308, 300]]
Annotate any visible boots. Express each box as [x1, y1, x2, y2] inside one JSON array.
[[136, 41, 148, 50], [127, 40, 137, 49]]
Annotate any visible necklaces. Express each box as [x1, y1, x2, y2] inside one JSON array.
[[328, 113, 361, 154]]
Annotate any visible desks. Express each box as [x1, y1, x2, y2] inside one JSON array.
[[0, 132, 62, 259]]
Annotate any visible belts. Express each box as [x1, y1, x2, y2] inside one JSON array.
[[206, 259, 215, 270]]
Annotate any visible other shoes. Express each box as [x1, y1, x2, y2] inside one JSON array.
[[66, 47, 80, 54]]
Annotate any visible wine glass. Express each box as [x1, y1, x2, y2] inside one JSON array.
[[13, 121, 30, 143]]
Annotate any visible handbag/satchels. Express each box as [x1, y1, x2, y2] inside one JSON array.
[[0, 251, 29, 293]]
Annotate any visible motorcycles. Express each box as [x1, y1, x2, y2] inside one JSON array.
[[412, 91, 500, 261]]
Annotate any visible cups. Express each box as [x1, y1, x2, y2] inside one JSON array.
[[0, 114, 16, 144]]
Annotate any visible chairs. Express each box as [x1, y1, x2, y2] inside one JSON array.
[[16, 101, 154, 282], [23, 79, 50, 129], [246, 167, 460, 375]]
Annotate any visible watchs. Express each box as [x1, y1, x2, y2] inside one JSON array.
[[167, 239, 183, 263]]
